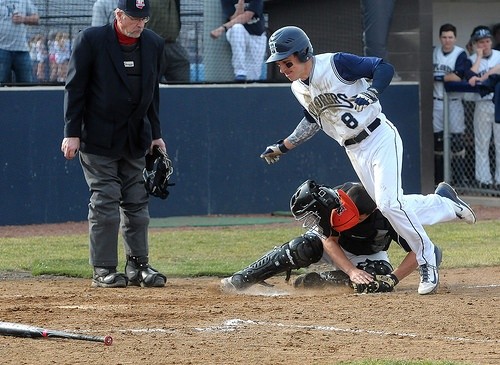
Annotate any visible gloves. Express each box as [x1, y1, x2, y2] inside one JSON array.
[[260, 139, 289, 164], [348, 88, 379, 112]]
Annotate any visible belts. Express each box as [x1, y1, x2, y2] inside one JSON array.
[[434, 96, 458, 102], [344, 117, 380, 146]]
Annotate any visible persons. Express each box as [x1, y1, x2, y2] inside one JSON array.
[[218, 180, 419, 293], [92, 0, 120, 27], [210, 0, 267, 79], [432, 23, 468, 189], [260, 26, 476, 295], [0, 0, 41, 82], [61, 0, 166, 288], [118, 0, 190, 82], [464, 25, 500, 189], [27, 31, 75, 82], [361, 0, 402, 80]]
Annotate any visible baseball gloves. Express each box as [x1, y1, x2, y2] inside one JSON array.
[[141, 142, 174, 199], [358, 274, 394, 293]]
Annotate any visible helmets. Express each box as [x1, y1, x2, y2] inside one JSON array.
[[265, 26, 314, 64]]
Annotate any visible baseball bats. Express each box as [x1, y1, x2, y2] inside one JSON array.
[[0, 322, 113, 344]]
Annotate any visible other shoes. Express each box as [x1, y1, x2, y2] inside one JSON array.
[[234, 75, 246, 82], [476, 180, 493, 189]]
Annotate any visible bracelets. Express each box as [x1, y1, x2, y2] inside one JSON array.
[[277, 140, 289, 153], [222, 25, 227, 32]]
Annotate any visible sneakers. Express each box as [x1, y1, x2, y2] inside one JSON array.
[[435, 183, 477, 224], [228, 269, 253, 294], [127, 272, 166, 286], [293, 271, 319, 287], [418, 247, 442, 295], [91, 272, 127, 287]]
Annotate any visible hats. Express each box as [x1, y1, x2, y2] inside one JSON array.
[[118, 0, 150, 17], [329, 190, 359, 232], [473, 30, 492, 41]]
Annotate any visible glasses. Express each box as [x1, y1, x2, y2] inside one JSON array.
[[119, 11, 150, 23]]
